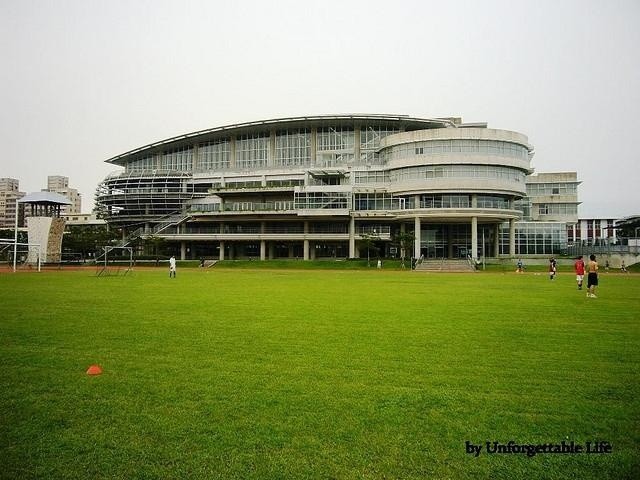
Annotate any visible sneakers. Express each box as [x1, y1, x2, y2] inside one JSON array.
[[591, 294, 597, 298], [578, 287, 582, 290], [587, 292, 591, 298]]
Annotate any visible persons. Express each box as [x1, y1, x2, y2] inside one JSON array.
[[574, 255, 586, 290], [548, 256, 557, 280], [169, 254, 176, 279], [586, 254, 598, 299], [200, 257, 206, 267], [604, 259, 610, 273], [515, 259, 525, 273], [620, 260, 627, 272]]
